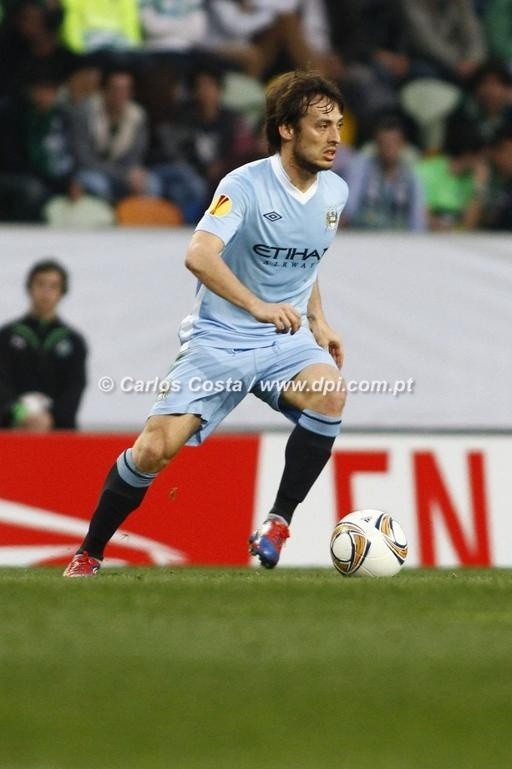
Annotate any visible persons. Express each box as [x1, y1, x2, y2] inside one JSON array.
[[0, 257, 86, 433], [61, 69, 348, 578]]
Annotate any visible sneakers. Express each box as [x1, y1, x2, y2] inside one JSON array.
[[247, 512, 290, 570], [62, 554, 102, 577]]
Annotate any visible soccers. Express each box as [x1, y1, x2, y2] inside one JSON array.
[[328, 509, 407, 579]]
